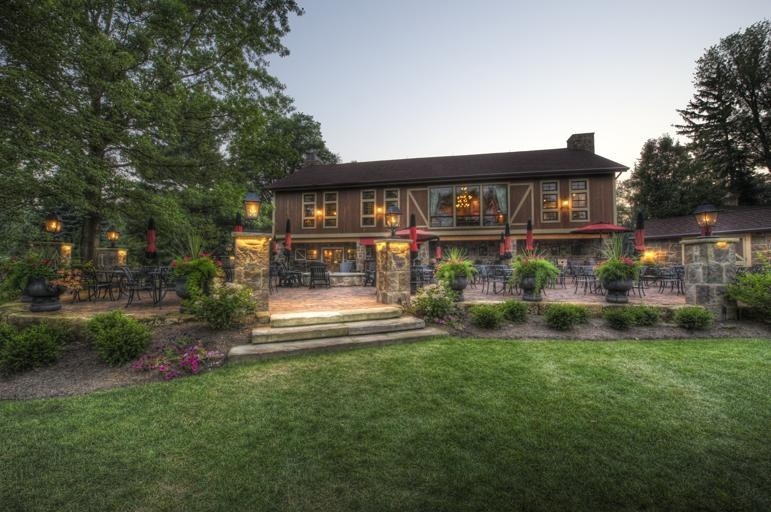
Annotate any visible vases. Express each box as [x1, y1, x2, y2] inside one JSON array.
[[26, 272, 65, 311]]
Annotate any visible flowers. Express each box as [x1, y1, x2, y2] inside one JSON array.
[[24, 249, 66, 278], [165, 229, 226, 283]]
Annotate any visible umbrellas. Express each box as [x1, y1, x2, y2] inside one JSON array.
[[526, 220, 535, 252], [434, 239, 443, 264], [272, 234, 278, 257], [504, 221, 513, 260], [234, 212, 243, 231], [409, 212, 419, 267], [285, 218, 293, 270], [573, 221, 631, 260], [499, 232, 506, 261], [145, 217, 156, 260], [635, 212, 646, 252]]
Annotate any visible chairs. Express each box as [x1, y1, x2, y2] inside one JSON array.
[[411, 262, 434, 293], [71, 261, 333, 314], [549, 258, 606, 295], [362, 259, 376, 287], [626, 262, 686, 298], [474, 262, 520, 295]]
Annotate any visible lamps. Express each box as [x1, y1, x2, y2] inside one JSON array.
[[383, 203, 402, 238], [693, 203, 721, 236], [105, 224, 121, 248], [240, 191, 264, 231], [45, 213, 63, 242]]
